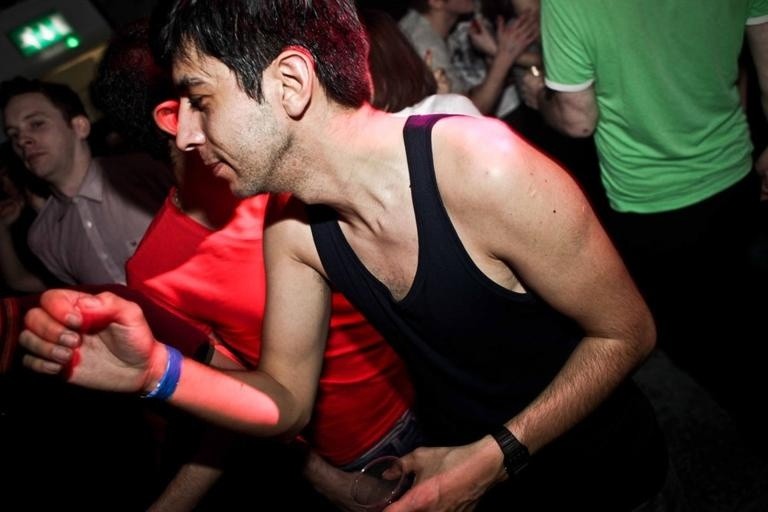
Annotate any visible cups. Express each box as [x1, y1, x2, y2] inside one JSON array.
[[350, 456, 412, 512]]
